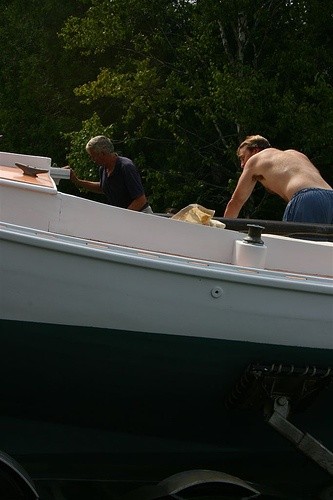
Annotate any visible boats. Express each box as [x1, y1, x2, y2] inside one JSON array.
[[0, 152, 333, 500]]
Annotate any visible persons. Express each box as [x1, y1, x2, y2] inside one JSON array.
[[222, 135, 333, 227], [61, 135, 152, 214]]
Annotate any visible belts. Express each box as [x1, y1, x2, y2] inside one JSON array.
[[139, 203, 149, 212]]
